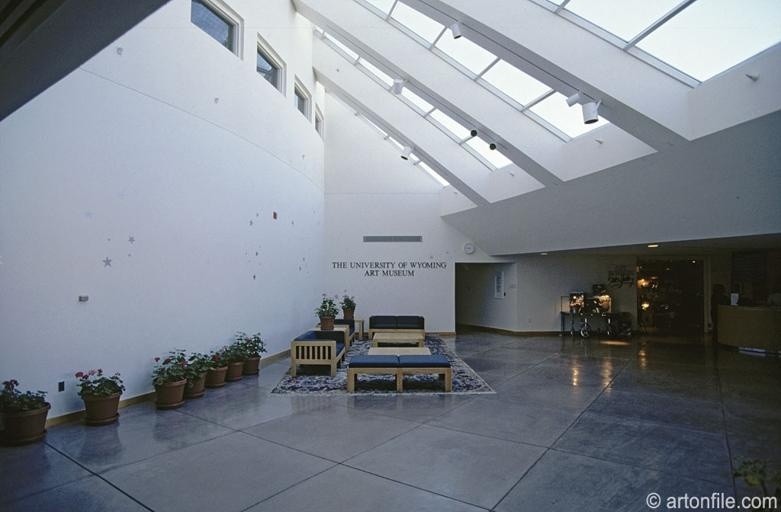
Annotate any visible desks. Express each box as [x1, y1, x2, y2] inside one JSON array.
[[355, 319, 365, 340], [370, 333, 432, 355]]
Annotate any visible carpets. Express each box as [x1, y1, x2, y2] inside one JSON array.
[[269, 336, 497, 395]]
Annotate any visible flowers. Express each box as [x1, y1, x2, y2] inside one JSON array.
[[152, 330, 269, 388], [339, 290, 357, 311], [74, 370, 126, 400], [0, 379, 48, 412], [314, 294, 340, 316]]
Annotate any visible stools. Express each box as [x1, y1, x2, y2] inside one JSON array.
[[348, 355, 452, 393]]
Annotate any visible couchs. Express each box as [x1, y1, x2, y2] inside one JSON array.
[[369, 315, 425, 338]]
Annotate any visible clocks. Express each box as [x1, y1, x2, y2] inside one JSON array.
[[463, 242, 476, 255]]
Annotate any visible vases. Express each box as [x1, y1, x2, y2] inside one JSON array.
[[153, 355, 261, 407], [342, 306, 355, 319], [81, 391, 122, 424], [319, 315, 336, 330], [1, 403, 52, 445]]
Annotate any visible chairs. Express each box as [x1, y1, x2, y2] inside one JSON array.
[[290, 318, 356, 377]]
